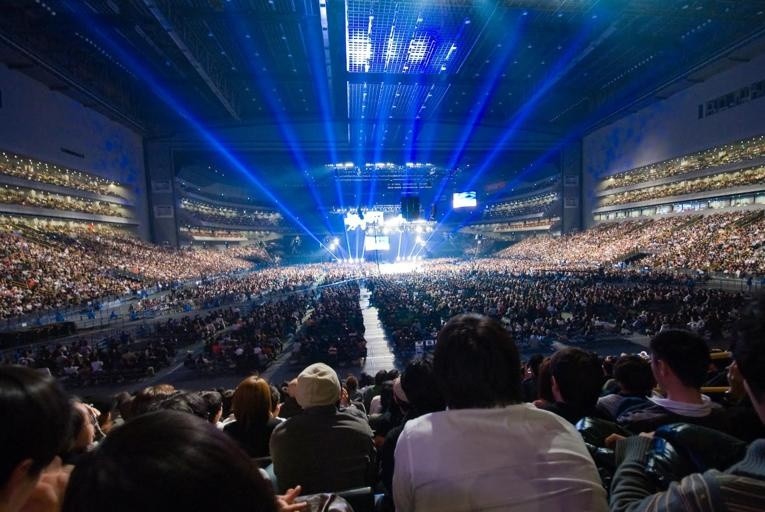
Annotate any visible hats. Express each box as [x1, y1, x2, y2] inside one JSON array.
[[394, 375, 411, 405], [294, 361, 342, 408]]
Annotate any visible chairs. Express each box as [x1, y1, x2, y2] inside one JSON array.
[[0, 284, 765, 512]]
